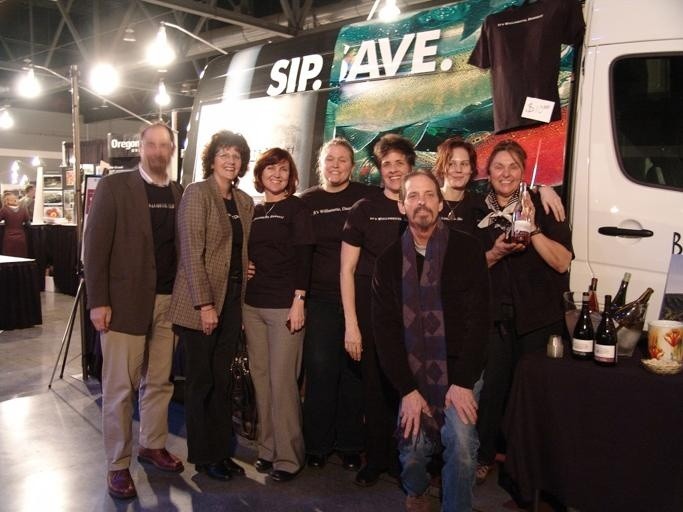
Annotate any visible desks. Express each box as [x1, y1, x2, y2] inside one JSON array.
[[0, 254, 43, 330], [504, 336, 683, 512], [29, 223, 77, 276]]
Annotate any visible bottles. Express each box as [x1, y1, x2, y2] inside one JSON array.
[[511, 180, 531, 247], [570, 274, 654, 359]]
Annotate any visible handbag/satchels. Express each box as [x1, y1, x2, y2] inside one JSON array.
[[230, 354, 257, 413]]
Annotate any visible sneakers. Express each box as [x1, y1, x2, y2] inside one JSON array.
[[406, 490, 431, 512], [474, 461, 492, 482]]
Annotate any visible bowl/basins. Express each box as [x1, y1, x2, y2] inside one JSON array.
[[564, 291, 648, 358]]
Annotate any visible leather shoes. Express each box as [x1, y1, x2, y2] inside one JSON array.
[[255, 458, 295, 482], [196, 456, 245, 481], [107, 467, 138, 498], [308, 451, 402, 485], [138, 445, 183, 471]]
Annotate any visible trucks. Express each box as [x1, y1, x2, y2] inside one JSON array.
[[183, 1, 683, 333]]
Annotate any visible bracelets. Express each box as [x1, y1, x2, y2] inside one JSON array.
[[294, 294, 306, 303], [535, 182, 548, 193], [200, 306, 215, 312], [528, 227, 540, 237]]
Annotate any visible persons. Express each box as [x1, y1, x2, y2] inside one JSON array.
[[241, 147, 314, 481], [0, 194, 29, 256], [339, 133, 420, 489], [369, 168, 490, 512], [17, 185, 34, 223], [83, 123, 185, 501], [246, 138, 368, 469], [165, 129, 255, 481], [464, 140, 575, 484], [432, 137, 566, 244]]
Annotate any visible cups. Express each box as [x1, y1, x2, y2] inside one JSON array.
[[547, 333, 566, 358], [649, 321, 683, 364]]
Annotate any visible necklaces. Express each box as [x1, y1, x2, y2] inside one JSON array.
[[443, 191, 462, 220], [260, 200, 277, 220]]
